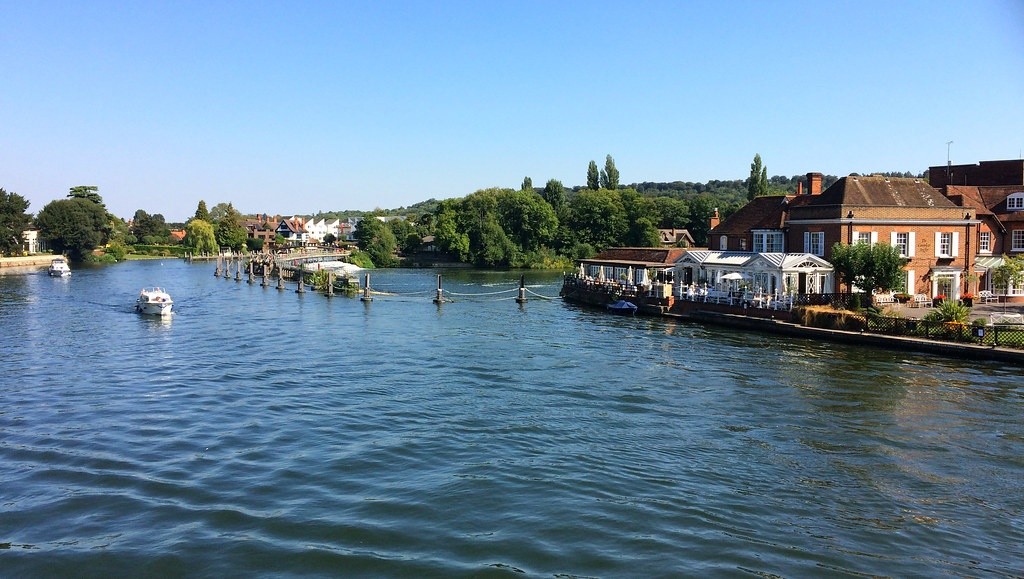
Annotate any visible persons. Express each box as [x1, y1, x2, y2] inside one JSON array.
[[596, 277, 770, 307]]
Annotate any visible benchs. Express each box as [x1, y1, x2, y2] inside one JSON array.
[[978, 290, 999, 305], [914, 294, 933, 309], [876, 296, 900, 306]]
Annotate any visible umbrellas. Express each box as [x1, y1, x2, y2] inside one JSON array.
[[721, 273, 751, 292]]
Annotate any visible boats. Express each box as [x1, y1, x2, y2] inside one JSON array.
[[48, 257, 72, 276], [606, 298, 638, 316], [136, 287, 174, 315]]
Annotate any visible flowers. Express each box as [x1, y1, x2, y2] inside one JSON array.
[[960, 292, 974, 299], [934, 295, 947, 299]]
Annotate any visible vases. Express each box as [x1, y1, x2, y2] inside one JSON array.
[[963, 298, 972, 307], [933, 299, 943, 308]]
